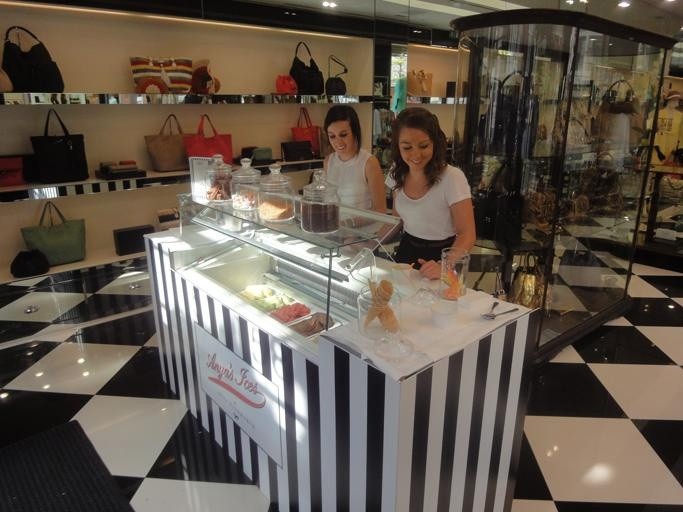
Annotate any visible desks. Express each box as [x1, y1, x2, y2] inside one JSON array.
[[634, 161, 683, 241]]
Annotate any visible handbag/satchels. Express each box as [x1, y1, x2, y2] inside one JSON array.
[[292, 126, 320, 151], [129, 57, 193, 94], [326, 77, 347, 94], [583, 152, 624, 215], [558, 250, 602, 301], [20, 201, 85, 266], [290, 41, 324, 94], [276, 76, 297, 94], [283, 141, 312, 161], [3, 27, 64, 93], [598, 79, 643, 145], [158, 206, 199, 232], [473, 252, 554, 309], [553, 101, 601, 144], [659, 176, 683, 199], [241, 146, 256, 158], [406, 69, 434, 97], [31, 107, 89, 184], [528, 191, 556, 224], [566, 195, 589, 221], [479, 72, 539, 157], [185, 114, 234, 168], [473, 190, 522, 246], [144, 114, 194, 171], [254, 147, 272, 161], [11, 249, 49, 277]]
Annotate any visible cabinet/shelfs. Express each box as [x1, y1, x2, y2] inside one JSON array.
[[142, 182, 543, 512], [388, 19, 580, 165], [0, 0, 373, 295]]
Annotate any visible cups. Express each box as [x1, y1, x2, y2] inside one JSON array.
[[440, 246, 469, 300], [430, 303, 458, 327]]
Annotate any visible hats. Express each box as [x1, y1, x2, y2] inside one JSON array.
[[194, 58, 220, 95], [664, 91, 682, 101]]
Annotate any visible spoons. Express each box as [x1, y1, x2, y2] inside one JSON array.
[[480, 302, 520, 320]]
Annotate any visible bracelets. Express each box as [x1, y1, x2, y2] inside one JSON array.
[[436, 260, 444, 265], [351, 217, 356, 230]]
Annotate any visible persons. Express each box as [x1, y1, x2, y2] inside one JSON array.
[[323, 105, 387, 246], [349, 107, 476, 280], [533, 124, 551, 157], [646, 90, 683, 165]]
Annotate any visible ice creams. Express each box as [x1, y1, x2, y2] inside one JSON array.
[[365, 280, 400, 333]]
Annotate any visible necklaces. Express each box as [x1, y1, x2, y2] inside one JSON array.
[[404, 172, 428, 199]]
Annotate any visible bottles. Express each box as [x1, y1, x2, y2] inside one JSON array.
[[300, 167, 343, 236], [227, 156, 261, 211], [256, 162, 295, 221], [205, 154, 234, 203], [357, 284, 404, 344]]
